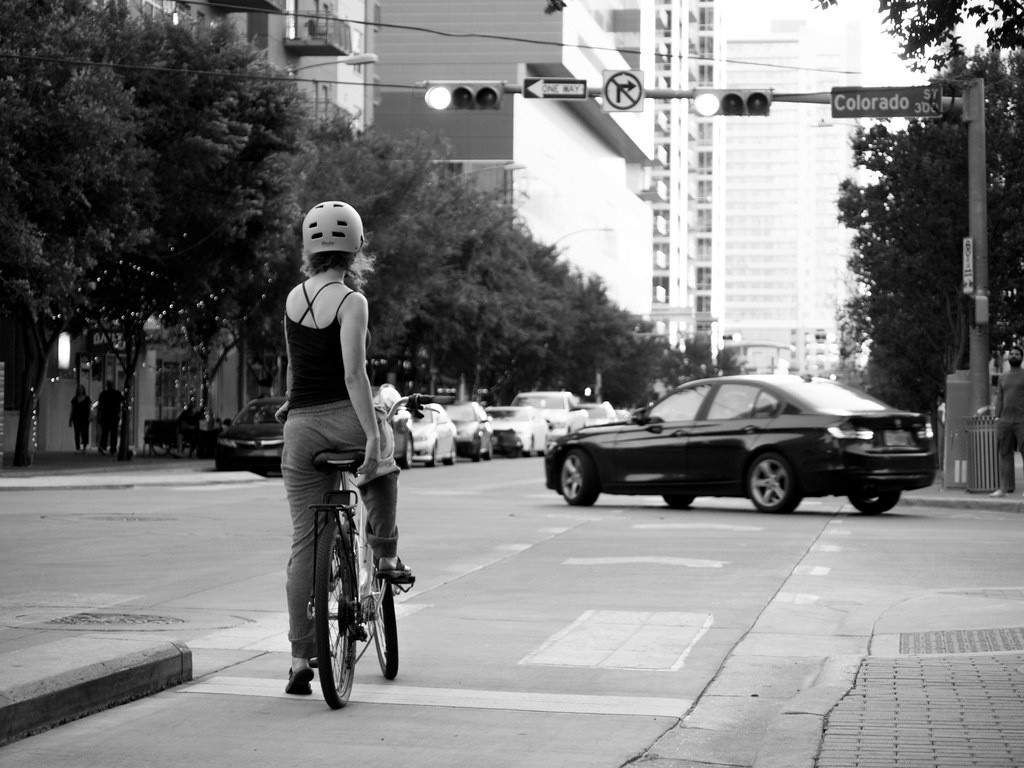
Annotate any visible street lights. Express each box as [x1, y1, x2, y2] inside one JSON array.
[[231, 50, 381, 414]]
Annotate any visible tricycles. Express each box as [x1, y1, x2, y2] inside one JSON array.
[[142, 416, 203, 460]]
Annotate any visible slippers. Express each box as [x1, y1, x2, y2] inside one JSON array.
[[376, 556, 411, 578], [285, 666, 314, 695]]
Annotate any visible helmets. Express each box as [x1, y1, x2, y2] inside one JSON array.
[[302, 200, 364, 253]]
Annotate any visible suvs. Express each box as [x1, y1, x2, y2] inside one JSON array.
[[372, 379, 415, 470]]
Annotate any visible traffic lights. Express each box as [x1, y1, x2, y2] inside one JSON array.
[[424, 79, 507, 112], [690, 85, 774, 118]]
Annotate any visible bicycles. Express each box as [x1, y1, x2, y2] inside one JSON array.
[[277, 391, 459, 711]]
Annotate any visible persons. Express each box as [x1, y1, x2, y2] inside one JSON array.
[[989, 346, 1024, 497], [97, 380, 122, 456], [69, 385, 92, 455], [176, 404, 196, 457], [275, 202, 417, 696]]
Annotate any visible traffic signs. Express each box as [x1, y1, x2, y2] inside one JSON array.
[[521, 75, 589, 102]]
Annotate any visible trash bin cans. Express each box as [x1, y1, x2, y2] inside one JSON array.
[[965, 414, 1016, 492]]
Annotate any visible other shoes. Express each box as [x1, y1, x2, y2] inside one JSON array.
[[989, 489, 1007, 497]]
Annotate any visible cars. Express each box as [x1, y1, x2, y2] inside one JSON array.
[[486, 387, 635, 457], [441, 400, 496, 463], [410, 403, 457, 468], [211, 396, 290, 477], [540, 373, 941, 519]]
